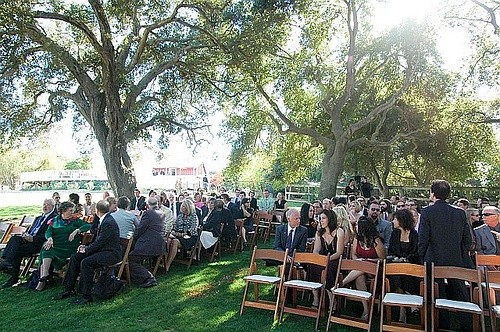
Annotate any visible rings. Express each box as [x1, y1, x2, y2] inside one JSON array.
[[48, 244, 49, 246]]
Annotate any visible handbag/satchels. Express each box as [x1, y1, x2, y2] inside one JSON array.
[[91, 268, 126, 300]]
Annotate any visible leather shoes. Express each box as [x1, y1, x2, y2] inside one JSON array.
[[53, 289, 75, 300], [139, 276, 157, 287], [69, 296, 93, 305]]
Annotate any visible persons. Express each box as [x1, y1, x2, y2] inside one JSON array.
[[273, 175, 500, 332], [1, 176, 288, 306]]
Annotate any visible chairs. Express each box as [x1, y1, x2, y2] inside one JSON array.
[[0, 205, 500, 332]]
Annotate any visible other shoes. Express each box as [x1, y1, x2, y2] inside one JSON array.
[[33, 284, 47, 291], [0, 277, 21, 289], [360, 313, 369, 320], [311, 302, 319, 309], [411, 308, 419, 314], [0, 258, 15, 273], [39, 274, 54, 282], [328, 305, 337, 315]]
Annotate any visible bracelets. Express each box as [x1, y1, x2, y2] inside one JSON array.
[[76, 229, 79, 232]]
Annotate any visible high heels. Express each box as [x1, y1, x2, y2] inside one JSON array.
[[399, 310, 407, 322]]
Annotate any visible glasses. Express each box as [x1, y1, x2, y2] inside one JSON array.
[[55, 196, 61, 199], [481, 213, 495, 216], [371, 208, 380, 212], [473, 214, 481, 217], [246, 202, 249, 204], [397, 205, 405, 208]]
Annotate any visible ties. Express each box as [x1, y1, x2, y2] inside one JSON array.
[[30, 216, 44, 236], [287, 230, 293, 248], [384, 212, 387, 220], [171, 203, 173, 211]]
